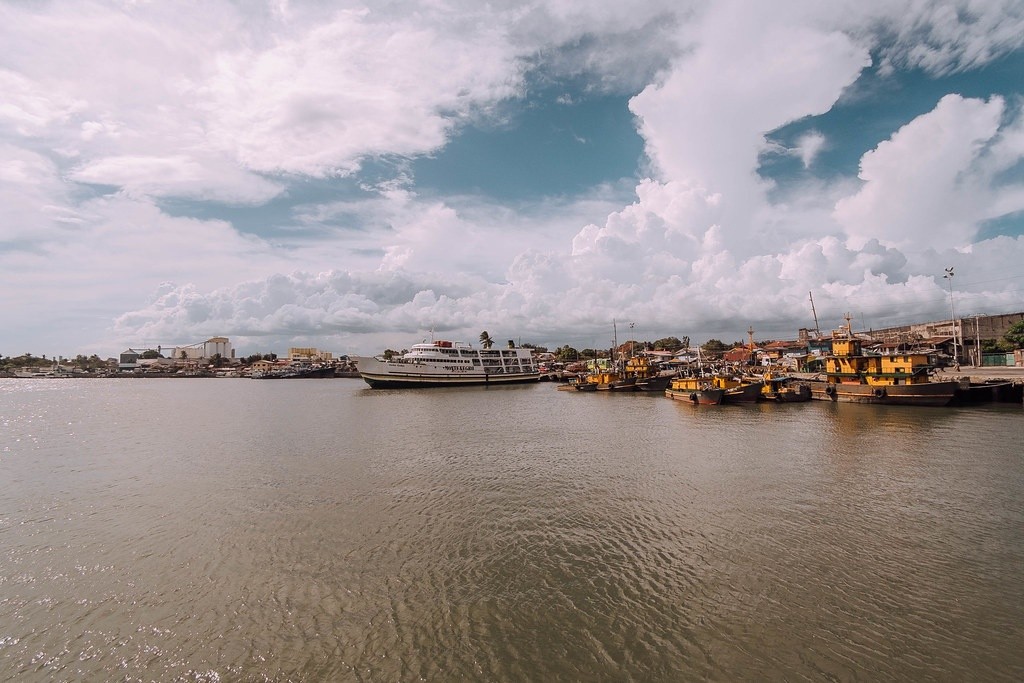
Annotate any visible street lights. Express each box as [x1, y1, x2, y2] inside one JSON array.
[[943, 267, 957, 363], [629, 323, 634, 356]]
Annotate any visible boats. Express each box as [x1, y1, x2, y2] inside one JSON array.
[[665, 369, 727, 405], [172, 361, 217, 378], [761, 374, 810, 403], [260, 371, 282, 379], [45, 365, 73, 379], [789, 338, 959, 406], [586, 365, 675, 392], [14, 367, 32, 378], [347, 330, 541, 389], [714, 374, 764, 400]]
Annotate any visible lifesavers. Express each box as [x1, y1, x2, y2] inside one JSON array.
[[690, 392, 696, 400], [608, 383, 613, 389], [826, 386, 834, 395], [874, 388, 886, 399]]
